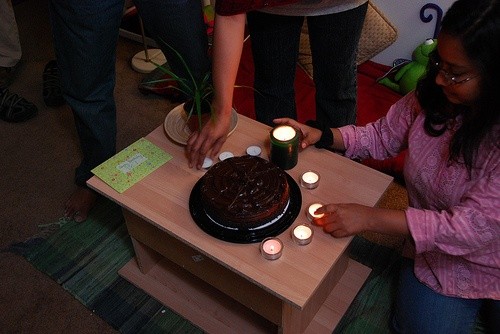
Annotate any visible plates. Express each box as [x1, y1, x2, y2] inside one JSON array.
[[189, 171, 302, 243]]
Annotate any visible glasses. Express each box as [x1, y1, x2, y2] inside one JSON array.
[[435, 59, 478, 87]]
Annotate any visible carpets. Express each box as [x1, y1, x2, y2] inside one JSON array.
[[3, 194, 489, 334]]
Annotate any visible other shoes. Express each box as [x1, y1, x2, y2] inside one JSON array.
[[42, 59, 66, 107], [0, 89, 39, 123]]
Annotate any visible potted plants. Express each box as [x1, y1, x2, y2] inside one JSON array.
[[141, 37, 266, 133]]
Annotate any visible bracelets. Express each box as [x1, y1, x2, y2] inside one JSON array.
[[307, 119, 334, 148]]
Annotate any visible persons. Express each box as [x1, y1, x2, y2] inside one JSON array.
[[185, 0, 370, 169], [50, 0, 214, 222], [0, 0, 40, 123], [272, 0, 500, 334]]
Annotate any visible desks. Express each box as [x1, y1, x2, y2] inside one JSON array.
[[86, 113, 395, 333]]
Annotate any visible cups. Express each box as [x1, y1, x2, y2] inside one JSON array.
[[201, 156, 213, 169], [269, 124, 299, 170], [246, 145, 262, 156], [260, 237, 284, 260], [218, 151, 235, 161], [291, 223, 314, 246], [306, 201, 327, 222], [300, 169, 321, 190]]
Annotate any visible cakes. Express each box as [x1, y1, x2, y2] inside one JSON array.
[[197, 154, 290, 231]]
